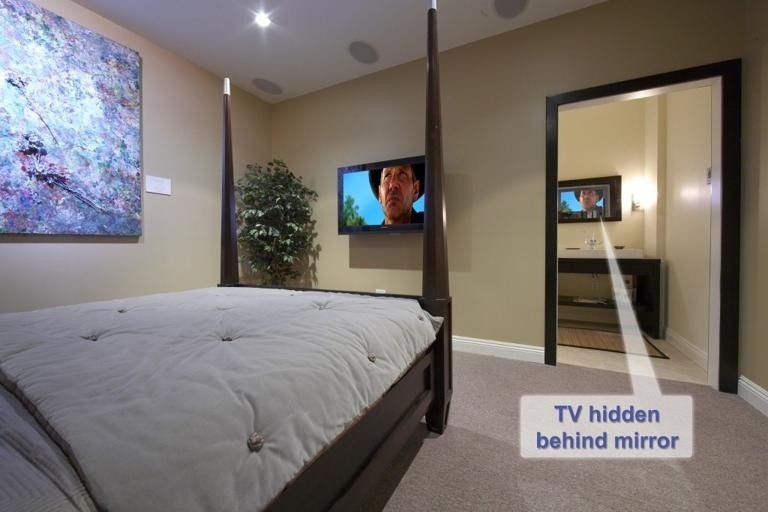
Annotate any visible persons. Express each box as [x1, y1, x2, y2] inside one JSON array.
[[369, 163, 424, 226], [569, 189, 605, 217]]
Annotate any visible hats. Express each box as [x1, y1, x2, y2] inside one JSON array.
[[369, 163, 425, 202], [575, 189, 603, 202]]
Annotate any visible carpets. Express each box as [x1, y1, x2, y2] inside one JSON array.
[[556, 325, 670, 359], [382, 349, 767, 512]]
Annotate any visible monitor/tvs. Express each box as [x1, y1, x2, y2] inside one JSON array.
[[557, 178, 614, 223], [338, 155, 425, 234]]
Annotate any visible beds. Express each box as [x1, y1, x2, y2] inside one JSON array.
[[1, 0, 453, 511]]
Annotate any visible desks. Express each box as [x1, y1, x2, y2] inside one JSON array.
[[558, 249, 661, 340]]
